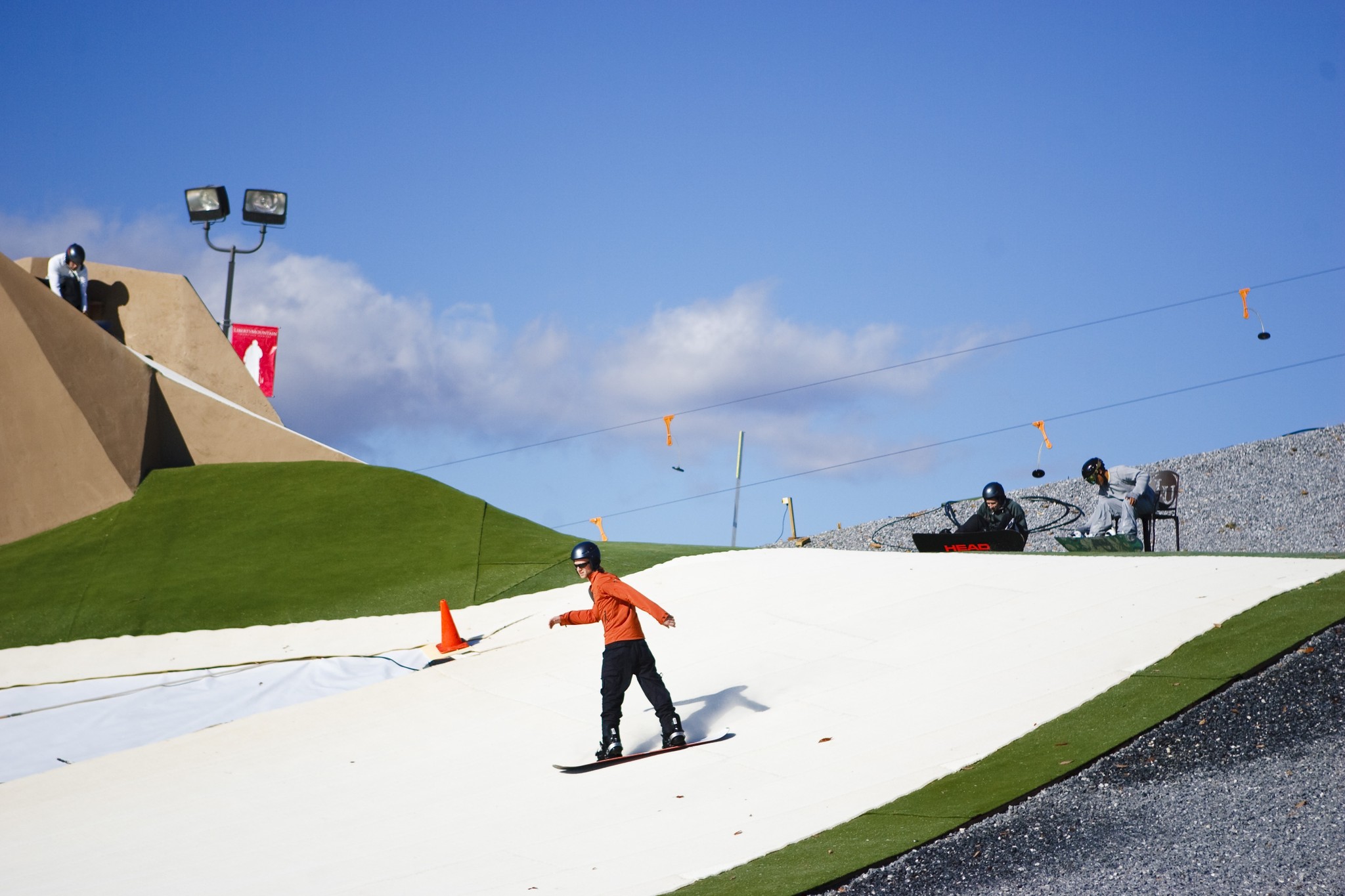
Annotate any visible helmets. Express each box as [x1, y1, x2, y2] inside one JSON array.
[[1081, 457, 1102, 482], [68, 243, 85, 263], [571, 542, 601, 571], [983, 482, 1005, 504]]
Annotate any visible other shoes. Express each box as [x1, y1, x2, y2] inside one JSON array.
[[661, 732, 685, 749], [595, 738, 622, 762]]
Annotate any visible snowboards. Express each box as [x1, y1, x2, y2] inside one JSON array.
[[553, 727, 730, 770], [1054, 534, 1143, 552], [912, 529, 1025, 552]]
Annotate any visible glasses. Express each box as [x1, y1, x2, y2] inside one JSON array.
[[1086, 474, 1096, 485], [574, 562, 590, 569]]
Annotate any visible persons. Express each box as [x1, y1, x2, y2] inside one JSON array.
[[938, 482, 1028, 551], [44, 243, 88, 314], [549, 541, 686, 761], [1071, 457, 1159, 538]]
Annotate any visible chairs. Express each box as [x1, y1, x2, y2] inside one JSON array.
[[1118, 470, 1181, 552]]
